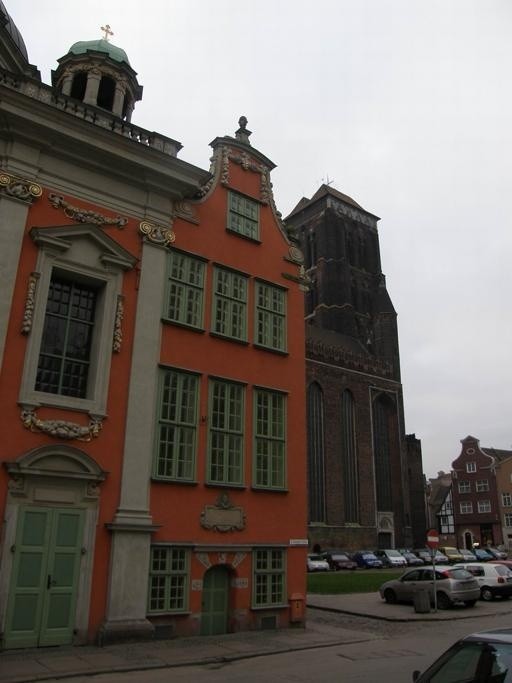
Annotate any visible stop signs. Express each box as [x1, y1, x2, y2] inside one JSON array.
[[426, 529, 442, 549]]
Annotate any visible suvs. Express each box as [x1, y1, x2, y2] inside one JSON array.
[[379, 563, 481, 609], [450, 561, 512, 601]]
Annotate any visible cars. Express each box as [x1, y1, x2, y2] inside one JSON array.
[[487, 558, 512, 572], [306, 546, 508, 573], [411, 625, 511, 682]]
